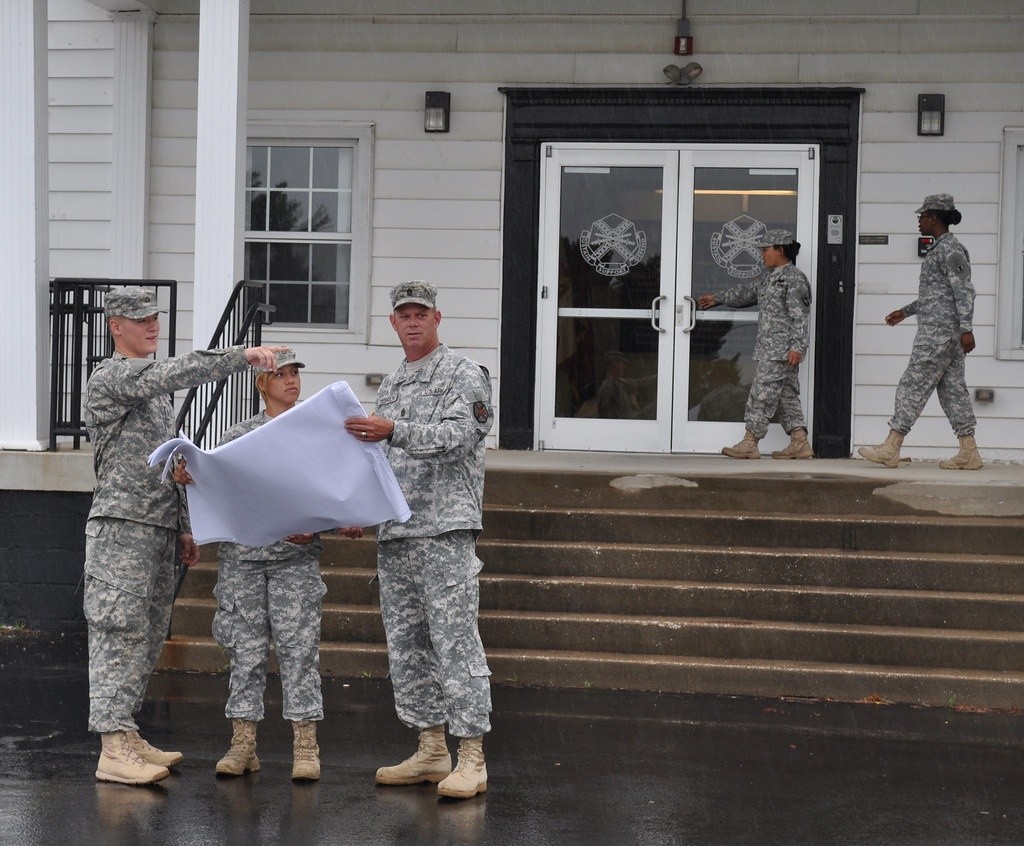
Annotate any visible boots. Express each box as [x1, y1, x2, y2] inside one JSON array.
[[95, 730, 170, 784], [376, 726, 453, 784], [939, 435, 983, 469], [858, 427, 904, 469], [215, 717, 262, 775], [126, 730, 183, 767], [291, 720, 322, 779], [721, 430, 762, 459], [771, 427, 815, 460], [438, 734, 487, 798]]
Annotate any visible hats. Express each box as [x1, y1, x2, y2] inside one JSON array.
[[389, 281, 438, 309], [914, 193, 955, 215], [104, 288, 168, 320], [755, 229, 794, 246], [255, 345, 306, 381]]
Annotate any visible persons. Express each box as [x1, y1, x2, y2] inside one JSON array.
[[699, 229, 814, 459], [83, 286, 289, 784], [596, 350, 659, 418], [858, 194, 983, 469], [174, 345, 363, 779], [689, 359, 748, 421], [340, 280, 495, 799]]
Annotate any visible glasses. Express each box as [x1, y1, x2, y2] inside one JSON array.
[[917, 216, 932, 220]]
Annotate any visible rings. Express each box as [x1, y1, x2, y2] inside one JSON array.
[[361, 432, 366, 438]]
